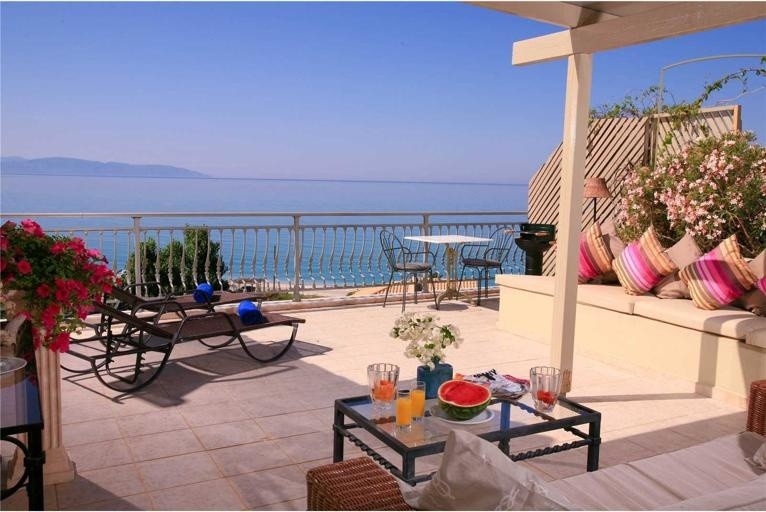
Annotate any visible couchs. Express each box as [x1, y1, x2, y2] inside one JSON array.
[[306, 380, 766, 511], [495, 243, 766, 399]]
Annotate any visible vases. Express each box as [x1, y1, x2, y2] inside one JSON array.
[[417, 363, 453, 399], [6, 307, 74, 494]]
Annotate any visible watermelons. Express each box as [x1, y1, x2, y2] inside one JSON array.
[[438, 380, 491, 419]]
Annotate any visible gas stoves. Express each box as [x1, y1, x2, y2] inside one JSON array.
[[516, 223, 556, 276]]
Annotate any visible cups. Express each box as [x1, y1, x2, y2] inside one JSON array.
[[529, 365, 561, 413], [409, 380, 426, 421], [395, 391, 412, 432], [365, 362, 399, 422]]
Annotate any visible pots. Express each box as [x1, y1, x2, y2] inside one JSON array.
[[505, 231, 551, 240]]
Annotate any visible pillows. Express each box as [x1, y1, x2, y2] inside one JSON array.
[[745, 441, 766, 470], [600, 217, 626, 282], [756, 277, 766, 295], [653, 231, 703, 299], [612, 226, 681, 296], [678, 234, 757, 311], [744, 440, 766, 475], [578, 221, 613, 283], [729, 250, 766, 315]]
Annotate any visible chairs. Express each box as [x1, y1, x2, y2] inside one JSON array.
[[456, 227, 515, 306], [380, 231, 440, 314]]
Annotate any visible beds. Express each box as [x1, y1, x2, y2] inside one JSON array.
[[61, 282, 305, 392]]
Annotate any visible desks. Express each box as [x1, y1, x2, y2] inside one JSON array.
[[0, 319, 44, 510], [404, 235, 494, 309]]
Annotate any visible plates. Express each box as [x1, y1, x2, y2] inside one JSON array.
[[428, 403, 495, 425]]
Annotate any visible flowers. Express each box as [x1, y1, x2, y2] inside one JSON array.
[[389, 311, 464, 370], [0, 219, 123, 354]]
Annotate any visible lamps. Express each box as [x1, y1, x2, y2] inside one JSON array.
[[584, 178, 613, 223]]
